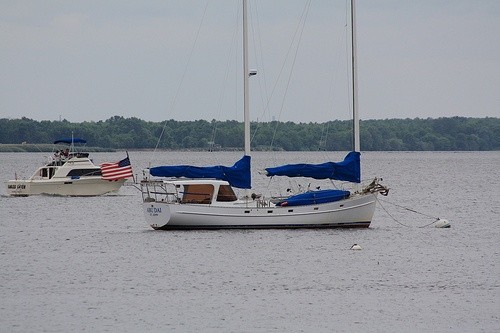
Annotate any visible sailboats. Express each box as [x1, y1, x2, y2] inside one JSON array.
[[139, 0, 390, 232]]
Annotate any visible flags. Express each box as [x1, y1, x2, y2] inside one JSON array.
[[101, 157, 133, 180]]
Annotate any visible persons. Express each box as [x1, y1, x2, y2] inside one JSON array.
[[54, 148, 69, 157]]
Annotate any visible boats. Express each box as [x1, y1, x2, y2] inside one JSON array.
[[2, 131, 130, 196]]
